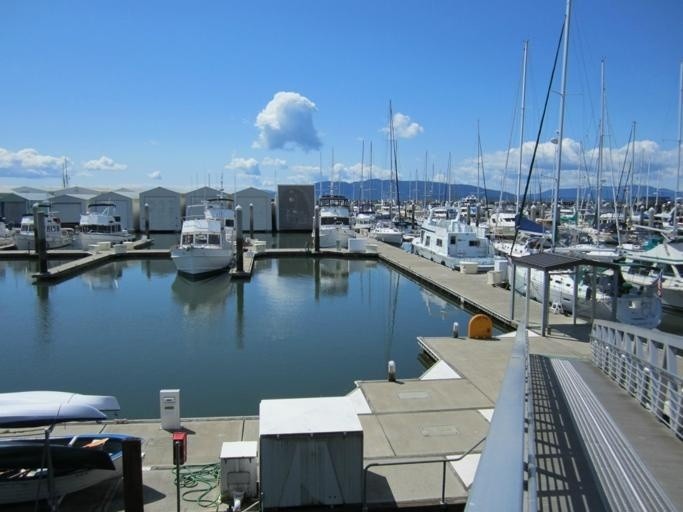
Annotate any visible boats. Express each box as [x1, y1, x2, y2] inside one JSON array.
[[0, 197, 136, 250], [171, 198, 236, 283], [312, 195, 356, 248]]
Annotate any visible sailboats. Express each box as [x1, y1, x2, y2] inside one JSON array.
[[352, 0, 683, 328]]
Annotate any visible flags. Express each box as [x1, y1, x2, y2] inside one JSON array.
[[652, 272, 663, 297]]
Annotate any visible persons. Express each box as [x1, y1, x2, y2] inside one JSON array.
[[583, 271, 591, 285]]
